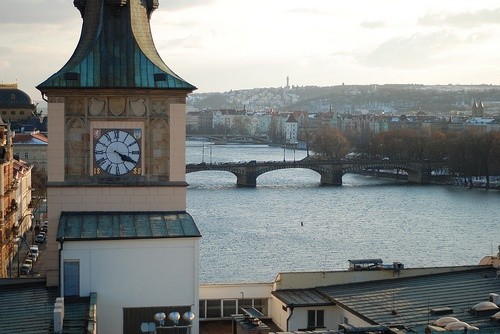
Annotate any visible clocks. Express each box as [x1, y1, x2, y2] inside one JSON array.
[[89, 121, 145, 178]]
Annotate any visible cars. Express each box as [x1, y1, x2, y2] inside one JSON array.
[[19, 220, 48, 273]]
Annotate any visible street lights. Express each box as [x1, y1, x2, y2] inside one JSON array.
[[201, 144, 213, 165], [283, 144, 296, 163]]
